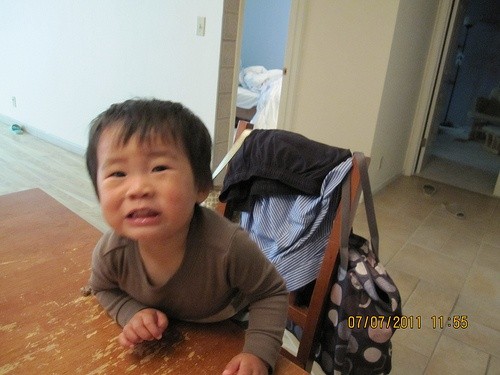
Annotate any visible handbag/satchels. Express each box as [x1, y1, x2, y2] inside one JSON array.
[[279, 153, 404, 375]]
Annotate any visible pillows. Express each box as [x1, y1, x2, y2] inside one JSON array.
[[471, 95, 499, 117]]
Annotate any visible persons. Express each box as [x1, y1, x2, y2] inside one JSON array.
[[85, 99, 290, 375]]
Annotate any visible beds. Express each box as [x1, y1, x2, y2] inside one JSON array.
[[236, 64, 283, 128]]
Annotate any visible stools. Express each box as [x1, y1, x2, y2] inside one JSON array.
[[481, 125, 500, 155]]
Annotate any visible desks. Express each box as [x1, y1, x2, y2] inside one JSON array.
[[0, 187, 311, 374]]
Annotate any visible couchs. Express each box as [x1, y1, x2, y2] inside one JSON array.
[[467, 87, 500, 143]]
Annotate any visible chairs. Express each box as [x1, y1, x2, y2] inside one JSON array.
[[215, 121, 371, 374]]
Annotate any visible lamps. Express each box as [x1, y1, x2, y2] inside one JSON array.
[[438, 15, 474, 127]]
